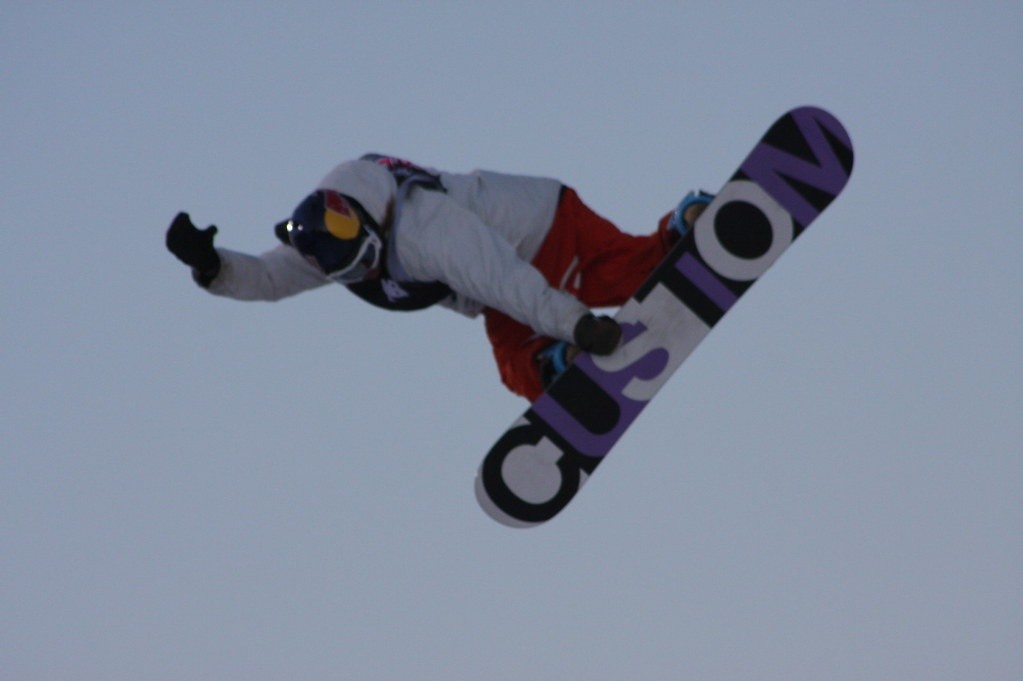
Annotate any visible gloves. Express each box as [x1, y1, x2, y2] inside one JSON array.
[[166, 212, 219, 271], [574, 314, 621, 355]]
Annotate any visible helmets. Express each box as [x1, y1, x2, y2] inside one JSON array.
[[287, 191, 364, 272]]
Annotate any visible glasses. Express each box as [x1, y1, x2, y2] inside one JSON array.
[[328, 230, 384, 287]]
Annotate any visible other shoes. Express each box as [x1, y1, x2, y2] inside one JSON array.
[[669, 191, 714, 232], [534, 341, 572, 386]]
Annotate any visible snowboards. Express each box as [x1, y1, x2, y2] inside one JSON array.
[[473, 102, 857, 532]]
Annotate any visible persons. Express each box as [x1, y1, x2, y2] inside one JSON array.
[[165, 152, 723, 402]]
